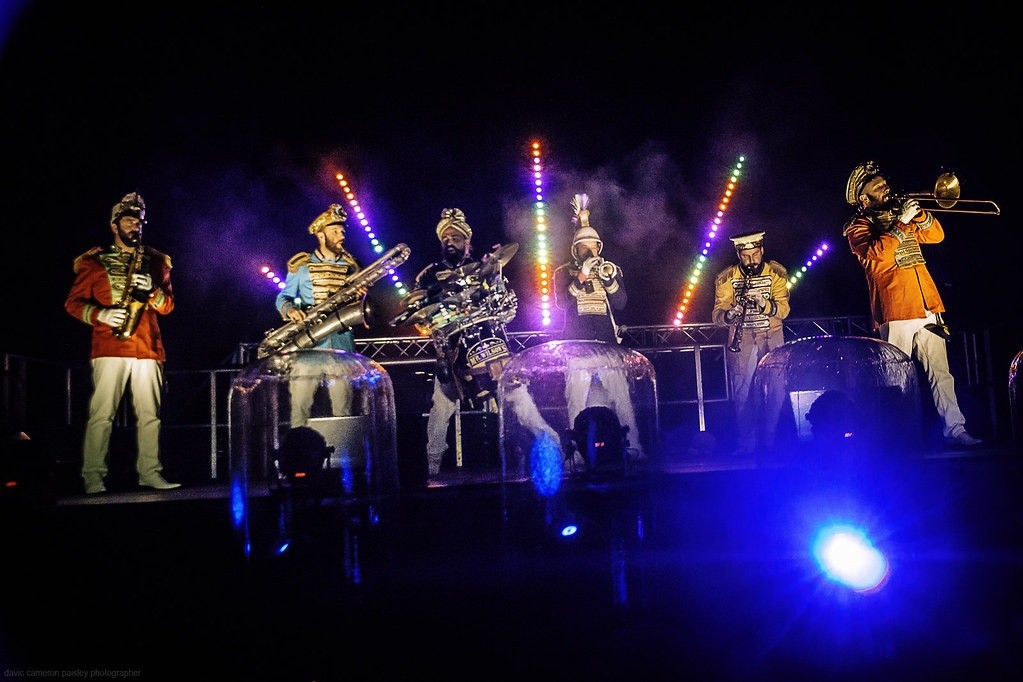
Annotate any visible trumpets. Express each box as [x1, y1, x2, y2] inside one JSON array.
[[593, 260, 619, 281]]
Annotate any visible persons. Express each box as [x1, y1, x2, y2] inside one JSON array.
[[276, 203, 368, 429], [843, 161, 983, 447], [712, 231, 791, 457], [64, 192, 181, 494], [554, 193, 647, 467], [414, 208, 562, 477]]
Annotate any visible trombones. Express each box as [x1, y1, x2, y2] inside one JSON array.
[[887, 171, 1001, 216]]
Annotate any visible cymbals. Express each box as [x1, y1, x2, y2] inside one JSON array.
[[387, 240, 520, 328]]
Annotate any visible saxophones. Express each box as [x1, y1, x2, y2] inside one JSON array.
[[111, 234, 149, 342], [727, 265, 754, 353], [257, 242, 412, 370]]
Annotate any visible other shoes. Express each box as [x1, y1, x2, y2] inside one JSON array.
[[732, 451, 753, 457]]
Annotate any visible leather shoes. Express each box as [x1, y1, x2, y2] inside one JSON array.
[[85, 479, 107, 494], [948, 433, 983, 449], [138, 472, 181, 490]]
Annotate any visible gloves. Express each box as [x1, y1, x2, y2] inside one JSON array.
[[581, 257, 602, 277], [97, 309, 126, 327], [132, 274, 152, 290]]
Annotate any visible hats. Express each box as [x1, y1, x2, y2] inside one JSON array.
[[436, 208, 472, 241], [729, 230, 765, 250], [846, 161, 880, 205], [112, 192, 146, 223], [308, 204, 347, 234], [570, 193, 604, 260]]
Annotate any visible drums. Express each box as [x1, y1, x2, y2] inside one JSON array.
[[442, 315, 522, 407]]
